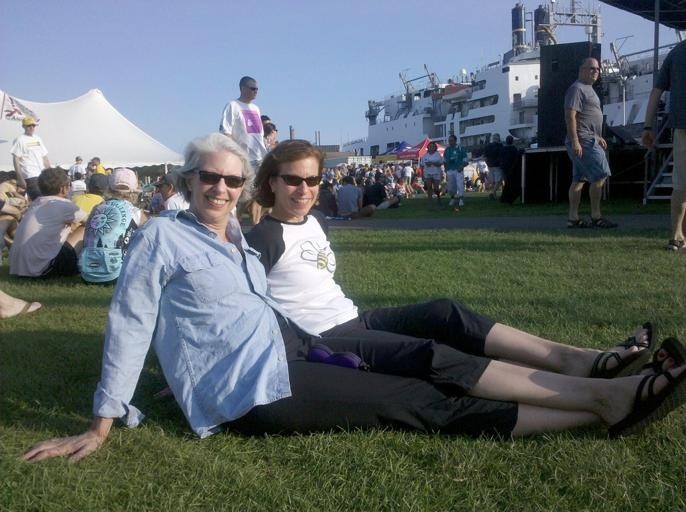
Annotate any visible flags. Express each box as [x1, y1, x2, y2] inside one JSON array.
[[1, 93, 40, 123]]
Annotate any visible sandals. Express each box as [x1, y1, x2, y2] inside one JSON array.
[[567, 219, 591, 229], [667, 236, 686, 252], [590, 216, 617, 228]]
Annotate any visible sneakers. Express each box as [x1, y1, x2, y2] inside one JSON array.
[[449, 198, 463, 206]]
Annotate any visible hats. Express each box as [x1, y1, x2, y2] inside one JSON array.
[[110, 169, 137, 191], [154, 175, 174, 187], [22, 117, 36, 126]]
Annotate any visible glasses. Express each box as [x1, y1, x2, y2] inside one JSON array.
[[198, 170, 245, 188], [279, 174, 322, 187]]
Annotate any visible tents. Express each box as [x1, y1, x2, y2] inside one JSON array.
[[375, 138, 446, 166], [1, 90, 185, 174]]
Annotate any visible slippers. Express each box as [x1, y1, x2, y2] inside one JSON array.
[[589, 322, 686, 438], [0, 300, 41, 320]]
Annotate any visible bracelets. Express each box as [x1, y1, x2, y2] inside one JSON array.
[[643, 126, 652, 131]]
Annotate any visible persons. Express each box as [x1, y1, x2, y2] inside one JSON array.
[[565, 57, 620, 227], [311, 160, 427, 221], [642, 38, 685, 253], [21, 132, 686, 468], [10, 115, 51, 201], [0, 155, 190, 287], [0, 289, 43, 321], [242, 137, 655, 378], [485, 133, 521, 206], [218, 75, 264, 226], [464, 159, 504, 192], [421, 135, 469, 207]]
